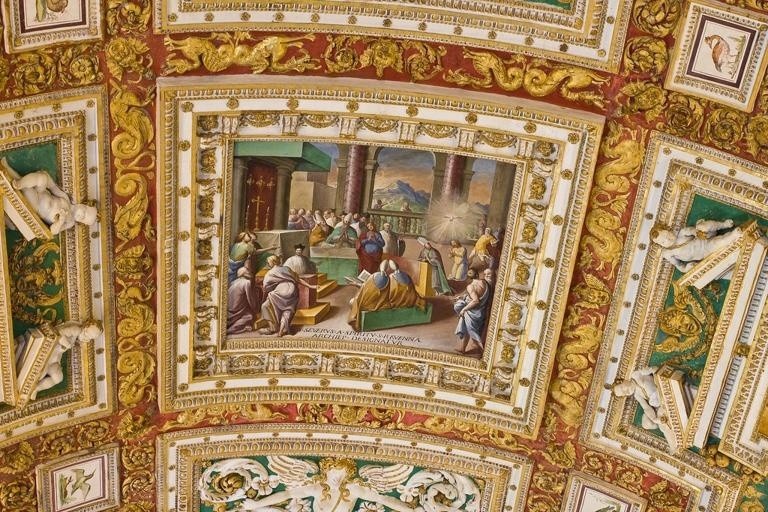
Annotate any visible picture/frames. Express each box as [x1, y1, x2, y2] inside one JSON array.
[[575, 129, 767, 511], [35, 442, 121, 512], [0, 0, 108, 55], [661, 0, 762, 115], [559, 470, 647, 512], [156, 422, 535, 512], [0, 82, 119, 447], [149, 0, 633, 75], [155, 74, 606, 442]]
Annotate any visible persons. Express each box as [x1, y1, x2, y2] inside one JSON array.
[[30, 318, 106, 403], [649, 217, 740, 274], [240, 455, 414, 512], [0, 154, 97, 238], [610, 366, 675, 456]]
